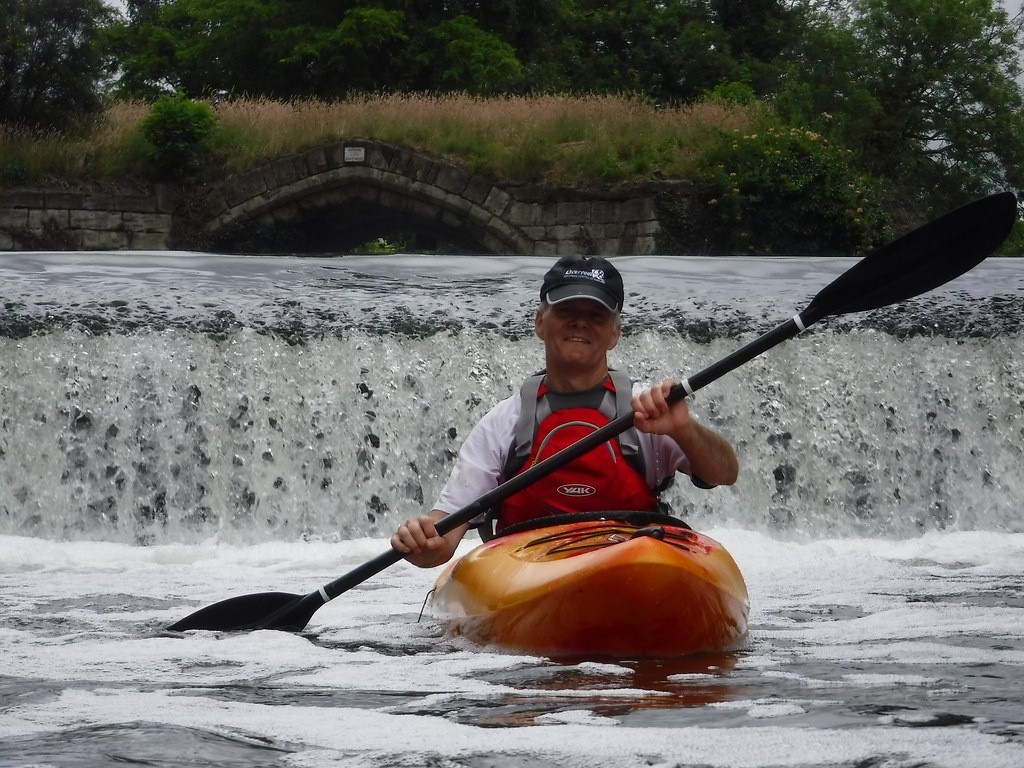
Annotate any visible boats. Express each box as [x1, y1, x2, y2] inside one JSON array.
[[427, 511, 750, 667]]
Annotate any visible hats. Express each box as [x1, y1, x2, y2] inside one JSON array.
[[540, 254, 624, 314]]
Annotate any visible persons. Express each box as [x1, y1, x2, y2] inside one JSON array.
[[391, 253, 740, 569]]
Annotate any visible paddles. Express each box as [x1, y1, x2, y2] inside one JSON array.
[[163, 186, 1018, 632]]
